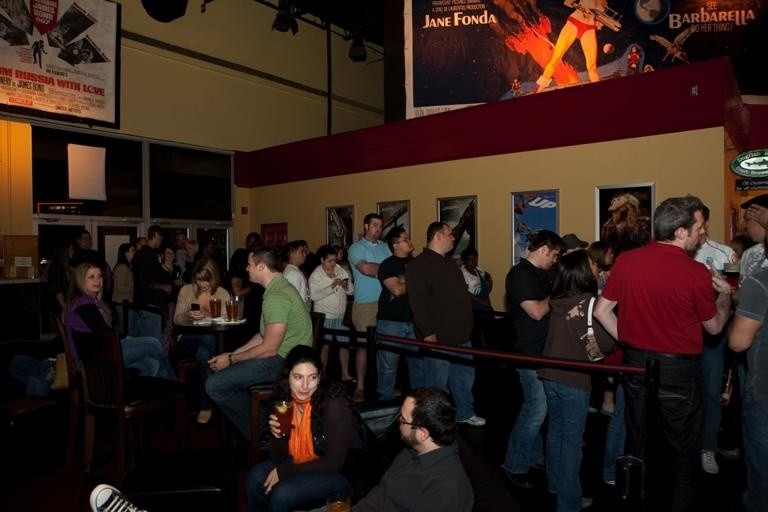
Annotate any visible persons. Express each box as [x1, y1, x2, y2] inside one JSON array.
[[587, 241, 612, 296], [178, 241, 202, 283], [309, 249, 356, 382], [734, 195, 768, 397], [595, 197, 732, 491], [299, 241, 316, 270], [64, 263, 175, 381], [133, 225, 165, 347], [39, 235, 71, 360], [729, 231, 768, 512], [136, 237, 146, 252], [89, 386, 474, 512], [376, 224, 421, 398], [32, 40, 47, 68], [205, 248, 314, 450], [333, 246, 354, 280], [557, 234, 589, 256], [627, 46, 642, 74], [511, 79, 524, 97], [601, 194, 650, 245], [283, 241, 311, 312], [154, 246, 184, 323], [720, 234, 753, 457], [243, 346, 380, 511], [349, 213, 402, 404], [112, 244, 137, 337], [407, 222, 485, 427], [232, 232, 265, 310], [684, 195, 738, 475], [460, 245, 493, 379], [504, 229, 564, 484], [534, 0, 607, 94], [173, 259, 232, 383], [737, 205, 768, 290], [67, 228, 113, 307], [537, 250, 614, 512], [173, 235, 190, 271]]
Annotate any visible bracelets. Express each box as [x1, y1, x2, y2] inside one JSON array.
[[226, 353, 236, 366], [227, 353, 237, 367]]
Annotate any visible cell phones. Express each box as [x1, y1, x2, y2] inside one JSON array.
[[338, 278, 348, 286], [190, 303, 199, 311]]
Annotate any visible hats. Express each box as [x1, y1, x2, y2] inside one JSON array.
[[560, 233, 591, 250], [742, 199, 767, 226]]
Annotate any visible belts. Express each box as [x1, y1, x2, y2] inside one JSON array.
[[624, 348, 702, 365]]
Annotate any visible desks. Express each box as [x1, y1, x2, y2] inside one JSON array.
[[0, 276, 48, 345]]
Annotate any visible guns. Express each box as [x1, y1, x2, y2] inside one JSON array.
[[446, 200, 475, 258], [329, 208, 350, 249]]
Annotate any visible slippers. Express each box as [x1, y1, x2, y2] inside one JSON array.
[[601, 408, 616, 418], [342, 376, 357, 384]]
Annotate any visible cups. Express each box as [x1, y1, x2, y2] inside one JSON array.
[[328, 498, 351, 511], [273, 401, 294, 437], [225, 300, 238, 323], [209, 296, 221, 320], [724, 263, 740, 289]]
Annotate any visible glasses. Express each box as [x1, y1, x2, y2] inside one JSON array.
[[393, 236, 413, 243], [396, 415, 433, 430]]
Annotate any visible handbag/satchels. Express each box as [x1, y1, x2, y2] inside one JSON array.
[[585, 296, 606, 363], [315, 393, 385, 488], [721, 364, 736, 406], [50, 352, 69, 389]]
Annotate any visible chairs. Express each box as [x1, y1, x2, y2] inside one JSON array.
[[3, 377, 60, 491], [71, 325, 172, 483], [120, 292, 173, 350], [237, 464, 280, 512], [248, 311, 328, 451], [173, 342, 224, 432]]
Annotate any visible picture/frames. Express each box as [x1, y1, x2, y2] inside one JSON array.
[[510, 188, 559, 270], [324, 204, 354, 245], [598, 185, 651, 251], [435, 194, 478, 265], [374, 199, 411, 241]]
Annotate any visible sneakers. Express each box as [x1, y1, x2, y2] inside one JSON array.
[[716, 446, 742, 459], [90, 485, 145, 512], [700, 449, 721, 474], [455, 415, 488, 427], [351, 392, 365, 403], [588, 405, 597, 412], [603, 477, 617, 486], [380, 389, 403, 401], [505, 471, 536, 489], [197, 409, 213, 423], [579, 495, 592, 510], [534, 74, 552, 92]]
